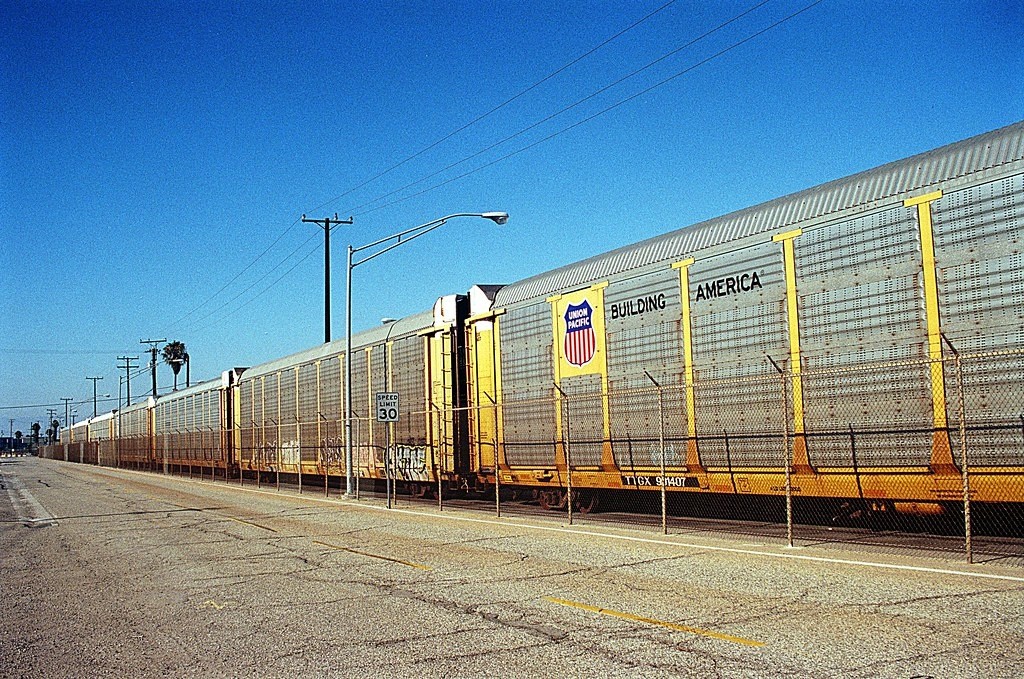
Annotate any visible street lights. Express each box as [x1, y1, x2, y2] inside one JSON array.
[[68, 394, 110, 441], [344, 210, 509, 497], [51, 410, 76, 442], [118, 358, 184, 466]]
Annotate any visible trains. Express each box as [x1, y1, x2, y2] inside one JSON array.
[[60, 120, 1024, 530]]
